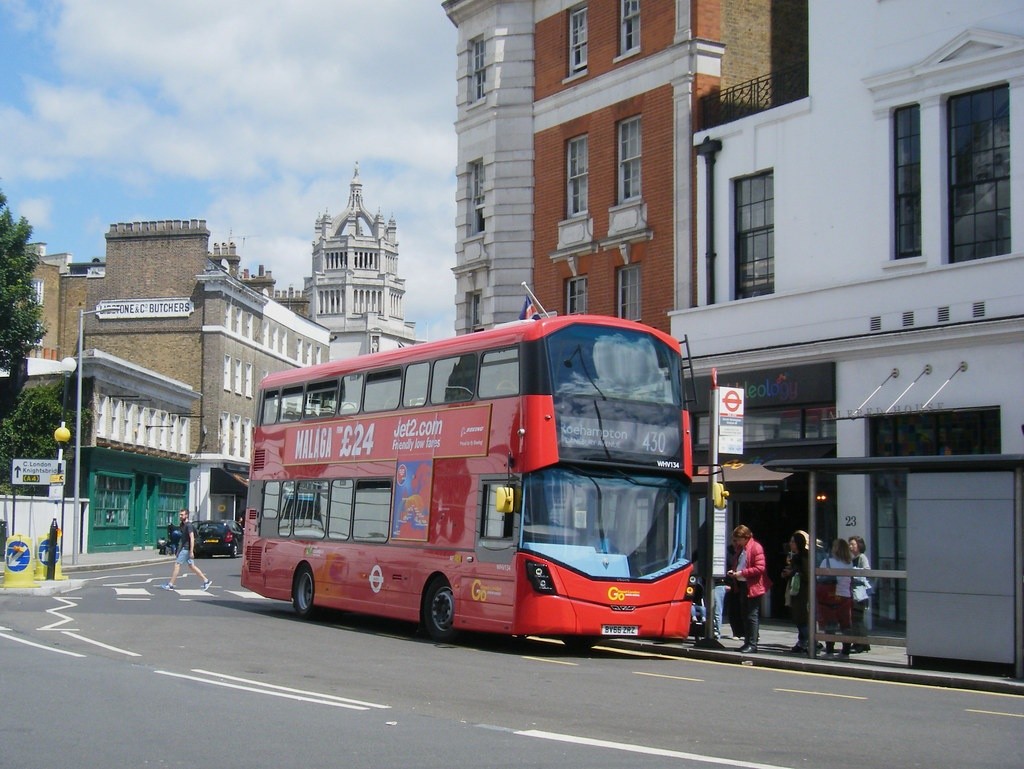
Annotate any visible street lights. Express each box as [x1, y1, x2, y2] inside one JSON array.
[[72, 306, 122, 564]]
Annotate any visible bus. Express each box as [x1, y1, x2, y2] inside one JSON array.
[[240, 314, 698, 649]]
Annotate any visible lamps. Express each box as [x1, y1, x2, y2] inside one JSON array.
[[563, 343, 607, 400]]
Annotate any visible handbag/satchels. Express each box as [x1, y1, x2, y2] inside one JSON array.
[[818, 557, 838, 584], [789, 572, 800, 596]]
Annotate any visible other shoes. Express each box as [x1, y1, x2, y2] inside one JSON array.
[[850, 643, 870, 652], [827, 650, 849, 659], [691, 615, 699, 623], [790, 643, 808, 654], [818, 650, 834, 659], [701, 616, 705, 622], [740, 644, 757, 653], [816, 643, 824, 653]]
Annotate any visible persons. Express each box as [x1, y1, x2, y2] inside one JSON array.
[[161, 509, 212, 591], [238, 516, 244, 528], [691, 525, 876, 653]]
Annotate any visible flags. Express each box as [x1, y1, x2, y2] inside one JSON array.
[[519, 294, 541, 320]]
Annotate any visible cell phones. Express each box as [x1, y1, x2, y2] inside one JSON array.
[[729, 572, 733, 575]]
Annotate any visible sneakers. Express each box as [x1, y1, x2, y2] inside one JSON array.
[[202, 580, 213, 590], [162, 584, 174, 591]]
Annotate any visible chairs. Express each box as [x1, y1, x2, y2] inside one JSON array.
[[285, 403, 356, 420]]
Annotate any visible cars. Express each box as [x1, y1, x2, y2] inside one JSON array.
[[194, 519, 244, 558]]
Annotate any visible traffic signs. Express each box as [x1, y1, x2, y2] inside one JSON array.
[[11, 458, 67, 486]]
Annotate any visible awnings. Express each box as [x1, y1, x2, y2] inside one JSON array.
[[210, 468, 248, 493], [691, 443, 835, 483]]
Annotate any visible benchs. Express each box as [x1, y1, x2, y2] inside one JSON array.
[[496, 379, 518, 395]]
